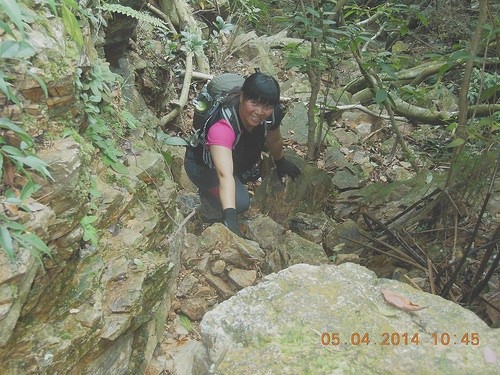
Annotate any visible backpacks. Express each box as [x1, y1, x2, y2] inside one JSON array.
[[193, 72, 276, 167]]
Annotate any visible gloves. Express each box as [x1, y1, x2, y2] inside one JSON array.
[[222, 208, 240, 235]]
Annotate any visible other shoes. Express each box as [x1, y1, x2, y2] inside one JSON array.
[[197, 188, 222, 221]]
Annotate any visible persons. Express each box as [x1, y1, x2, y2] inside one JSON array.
[[183, 73, 302, 237]]
